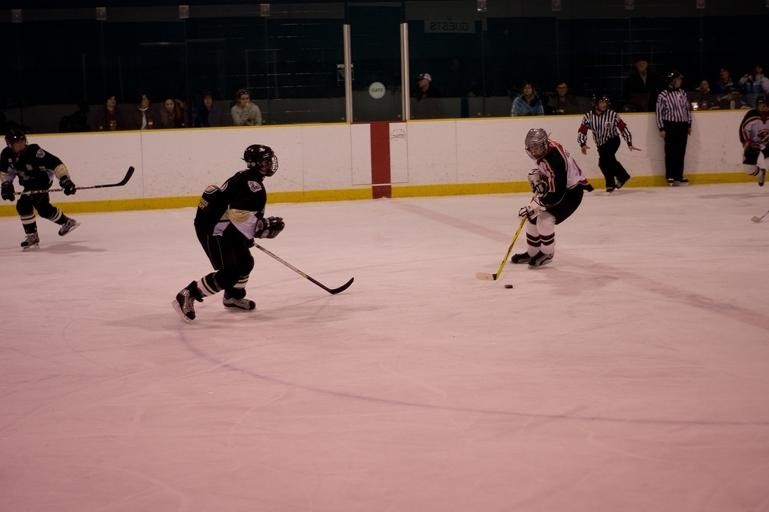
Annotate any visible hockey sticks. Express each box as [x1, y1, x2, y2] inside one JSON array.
[[751, 213, 768, 223], [253, 242, 354, 294], [476, 192, 538, 282], [12, 166, 134, 196]]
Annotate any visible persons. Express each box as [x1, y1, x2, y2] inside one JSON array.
[[97, 89, 231, 131], [738, 93, 769, 185], [577, 91, 643, 191], [624, 60, 768, 110], [409, 72, 442, 101], [512, 128, 593, 268], [230, 89, 262, 126], [511, 81, 577, 116], [176, 144, 285, 323], [0, 128, 76, 249], [655, 70, 694, 188]]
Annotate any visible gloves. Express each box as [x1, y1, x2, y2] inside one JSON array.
[[254, 215, 285, 238], [518, 197, 549, 221], [59, 176, 77, 195], [0, 182, 18, 201], [528, 173, 548, 193]]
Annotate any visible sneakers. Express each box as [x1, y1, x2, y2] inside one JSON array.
[[666, 177, 689, 185]]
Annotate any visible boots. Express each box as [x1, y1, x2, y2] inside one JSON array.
[[529, 250, 554, 266], [222, 289, 256, 311], [176, 280, 202, 321], [57, 218, 78, 236], [605, 174, 631, 193], [20, 234, 41, 247], [758, 167, 765, 187], [511, 252, 531, 263]]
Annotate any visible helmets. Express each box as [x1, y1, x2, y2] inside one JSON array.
[[667, 70, 686, 89], [755, 93, 769, 116], [3, 127, 29, 152], [589, 90, 612, 113], [241, 144, 280, 177], [524, 127, 549, 160]]
